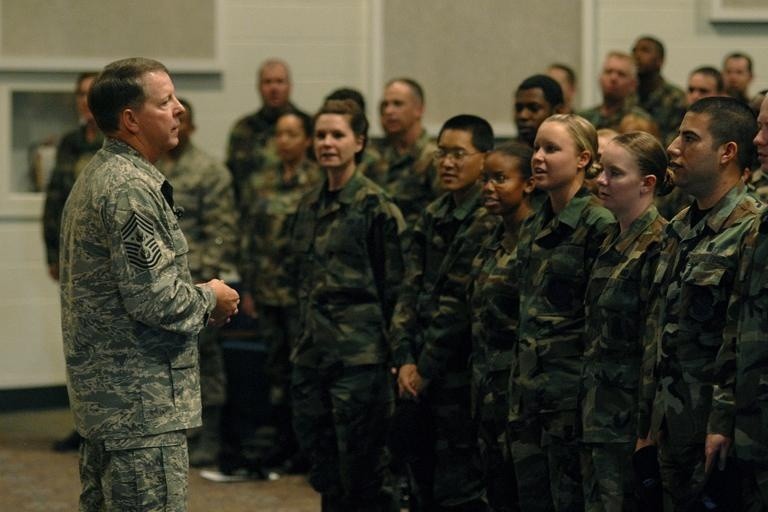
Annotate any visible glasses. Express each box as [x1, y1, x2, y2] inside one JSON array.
[[434, 150, 479, 159]]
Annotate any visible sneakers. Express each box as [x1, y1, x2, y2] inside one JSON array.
[[53, 429, 80, 451], [199, 467, 250, 483]]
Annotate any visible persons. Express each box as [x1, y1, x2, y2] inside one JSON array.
[[200, 33, 767, 510], [42, 69, 105, 454], [13, 140, 58, 194], [152, 97, 243, 473], [55, 57, 239, 511]]
